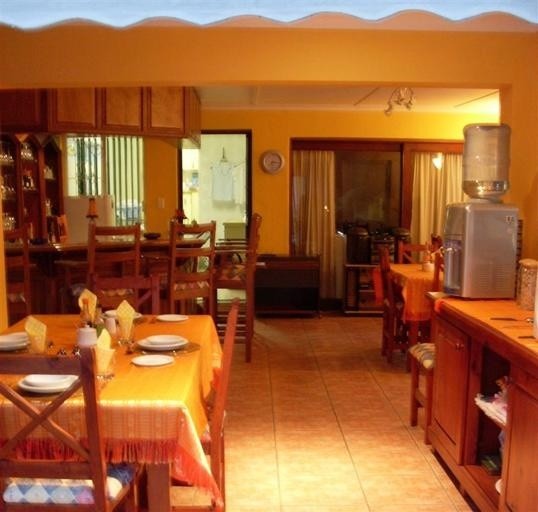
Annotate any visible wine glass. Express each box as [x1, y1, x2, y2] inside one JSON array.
[[0, 142, 57, 233]]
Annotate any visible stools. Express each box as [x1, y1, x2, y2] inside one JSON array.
[[408, 344, 434, 445]]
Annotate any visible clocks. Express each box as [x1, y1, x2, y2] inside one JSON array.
[[259, 149, 286, 175]]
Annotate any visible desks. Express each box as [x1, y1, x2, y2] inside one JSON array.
[[426, 292, 538, 512]]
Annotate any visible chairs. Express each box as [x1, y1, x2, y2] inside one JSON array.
[[1, 212, 263, 364], [378, 239, 444, 373], [147, 297, 241, 512], [0, 347, 143, 512]]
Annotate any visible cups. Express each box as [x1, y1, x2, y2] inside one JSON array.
[[117, 323, 137, 353], [106, 318, 116, 334], [90, 344, 116, 384], [79, 325, 97, 348]]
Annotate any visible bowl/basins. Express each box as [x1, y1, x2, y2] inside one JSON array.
[[30, 237, 49, 243], [144, 232, 163, 240]]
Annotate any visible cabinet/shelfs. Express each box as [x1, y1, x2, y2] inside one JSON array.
[[342, 264, 384, 318], [0, 89, 202, 244], [255, 254, 323, 318]]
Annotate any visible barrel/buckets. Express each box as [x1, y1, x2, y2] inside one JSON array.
[[462, 123, 511, 197]]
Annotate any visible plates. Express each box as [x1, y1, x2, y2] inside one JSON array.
[[131, 354, 174, 366], [0, 332, 29, 352], [105, 309, 141, 319], [156, 314, 189, 323], [137, 334, 188, 352], [17, 374, 80, 394]]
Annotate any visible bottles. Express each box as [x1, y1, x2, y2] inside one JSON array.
[[79, 298, 92, 327], [517, 258, 538, 311]]
